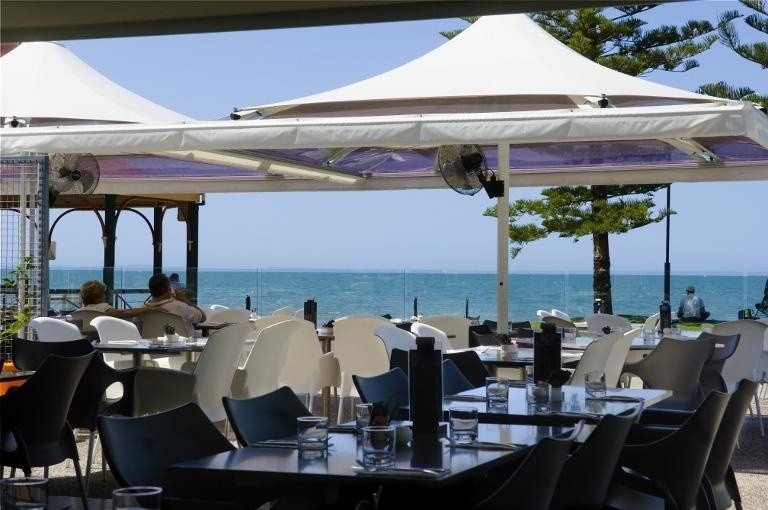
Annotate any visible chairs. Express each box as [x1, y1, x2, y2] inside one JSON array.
[[1, 302, 767, 510]]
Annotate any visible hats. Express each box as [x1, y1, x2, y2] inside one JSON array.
[[686, 285, 696, 292], [169, 272, 181, 281]]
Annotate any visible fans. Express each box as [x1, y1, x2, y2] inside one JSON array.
[[437, 144, 506, 200], [47, 152, 102, 203]]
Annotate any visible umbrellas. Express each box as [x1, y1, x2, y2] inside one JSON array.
[[0, 43, 210, 317], [232, 13, 762, 333]]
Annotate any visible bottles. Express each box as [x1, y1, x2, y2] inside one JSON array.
[[535, 323, 563, 377], [406, 335, 442, 441], [244, 296, 256, 318], [304, 296, 317, 329], [659, 300, 671, 329]]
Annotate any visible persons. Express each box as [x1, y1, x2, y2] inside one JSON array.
[[169, 273, 180, 283], [132, 272, 205, 342], [76, 279, 169, 321], [676, 284, 710, 322]]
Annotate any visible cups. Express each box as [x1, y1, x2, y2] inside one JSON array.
[[585, 369, 607, 397], [361, 427, 400, 469], [485, 402, 509, 416], [298, 451, 329, 471], [485, 375, 509, 401], [523, 364, 552, 404], [644, 326, 657, 342], [528, 405, 551, 416], [448, 407, 483, 444], [354, 432, 361, 449], [562, 326, 577, 343], [355, 400, 371, 431], [297, 414, 329, 452], [672, 325, 683, 336], [184, 335, 198, 345], [584, 397, 606, 412]]
